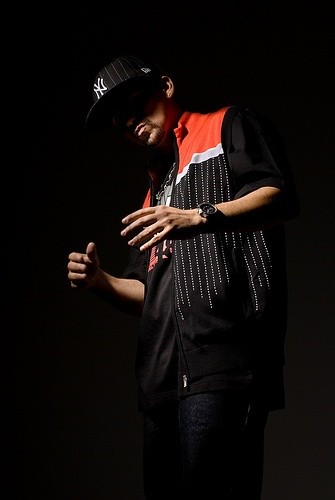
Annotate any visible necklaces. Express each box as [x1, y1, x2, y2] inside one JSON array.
[[155, 163, 177, 202]]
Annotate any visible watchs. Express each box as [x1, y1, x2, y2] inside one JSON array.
[[195, 202, 218, 229]]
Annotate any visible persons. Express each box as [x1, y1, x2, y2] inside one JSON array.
[[66, 56, 302, 500]]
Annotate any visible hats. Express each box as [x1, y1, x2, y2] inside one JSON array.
[[83, 54, 172, 126]]
